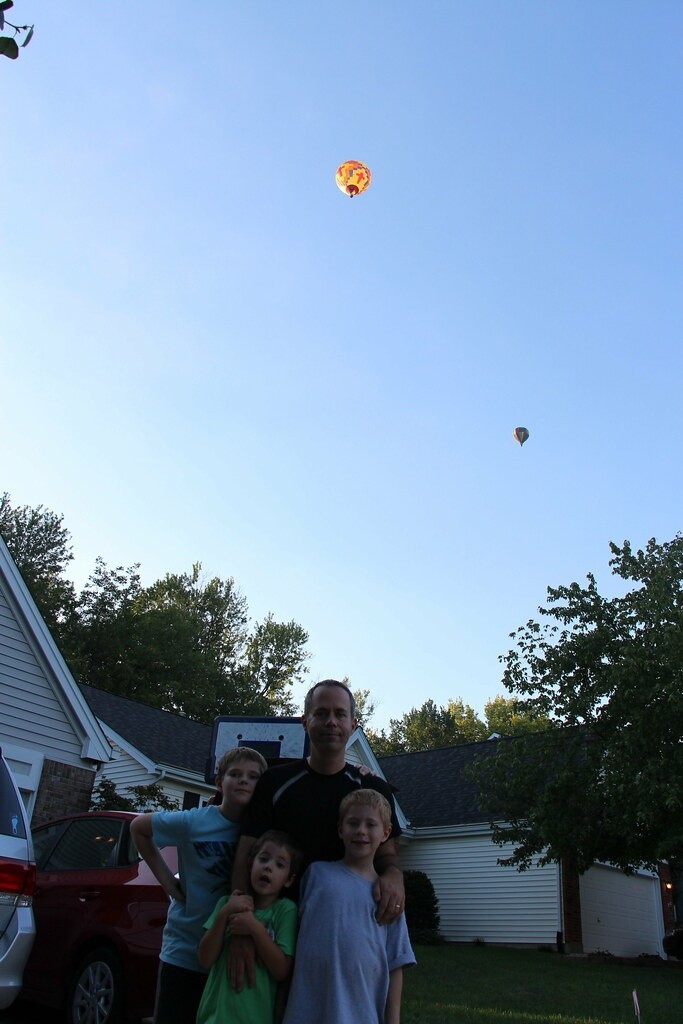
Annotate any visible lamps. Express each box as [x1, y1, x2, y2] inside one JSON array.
[[664, 881, 674, 906]]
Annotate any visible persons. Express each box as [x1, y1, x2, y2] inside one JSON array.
[[128, 746, 379, 1024], [274, 790, 418, 1024], [227, 680, 405, 993], [196, 832, 299, 1024]]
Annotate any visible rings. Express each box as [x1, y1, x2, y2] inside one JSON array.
[[394, 904, 401, 908]]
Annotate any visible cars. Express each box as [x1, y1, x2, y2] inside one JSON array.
[[27, 809, 180, 1024], [0, 746, 38, 1010]]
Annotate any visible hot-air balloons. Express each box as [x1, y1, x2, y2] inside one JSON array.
[[335, 160, 372, 200], [513, 427, 529, 447]]
